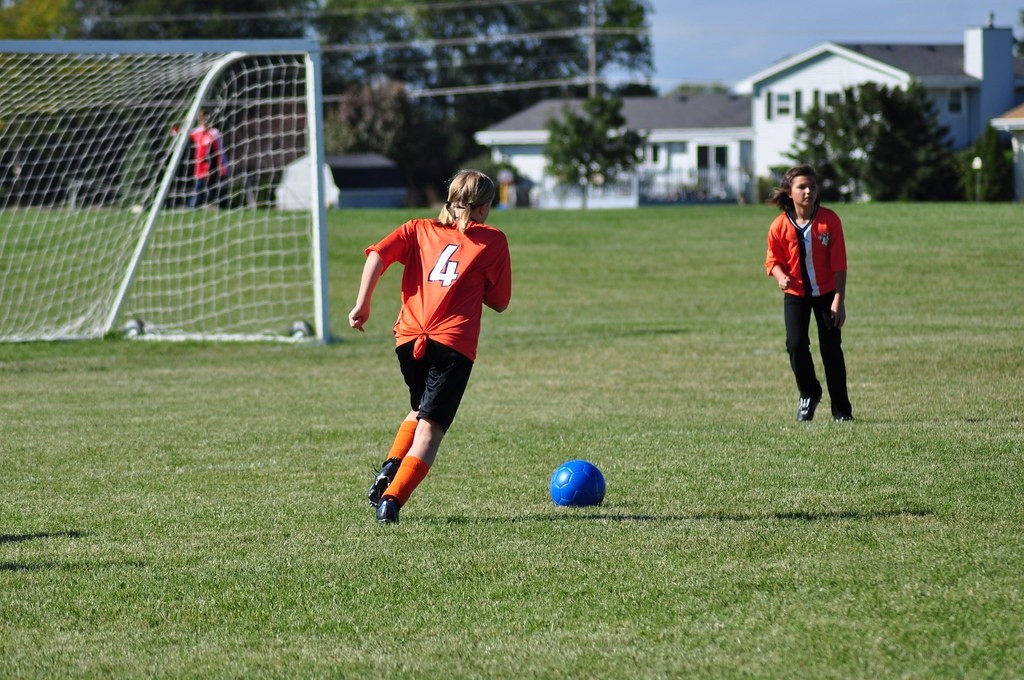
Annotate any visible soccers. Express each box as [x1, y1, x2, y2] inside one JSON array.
[[552, 458, 608, 509]]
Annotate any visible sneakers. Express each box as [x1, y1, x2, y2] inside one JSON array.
[[376, 499, 400, 524], [367, 461, 399, 508], [835, 414, 854, 423], [797, 388, 822, 423]]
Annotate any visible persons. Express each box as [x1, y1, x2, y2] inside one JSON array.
[[349, 171, 513, 524], [169, 106, 229, 208], [766, 165, 854, 421]]
